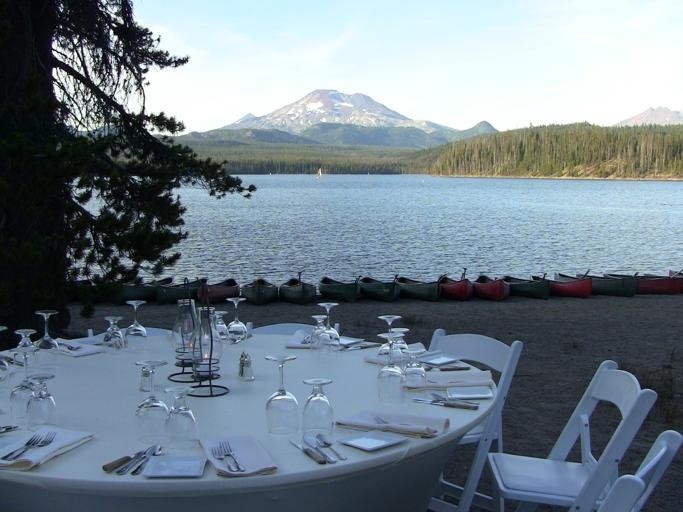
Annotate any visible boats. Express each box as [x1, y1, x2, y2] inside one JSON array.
[[531, 275, 592, 298], [474, 275, 510, 301], [318, 277, 360, 301], [361, 277, 400, 301], [603, 273, 681, 295], [157, 277, 208, 302], [503, 276, 550, 300], [439, 274, 472, 301], [198, 279, 240, 302], [575, 272, 636, 297], [279, 278, 315, 302], [397, 276, 440, 302], [242, 280, 278, 304], [667, 269, 682, 280], [115, 276, 175, 299]]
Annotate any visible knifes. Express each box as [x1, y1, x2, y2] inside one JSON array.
[[290, 440, 325, 465], [117, 444, 156, 475], [411, 397, 480, 410], [102, 448, 146, 473], [303, 436, 336, 465]]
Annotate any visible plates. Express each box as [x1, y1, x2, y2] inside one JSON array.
[[141, 454, 208, 477], [447, 385, 493, 399], [330, 334, 362, 345], [420, 354, 457, 366], [338, 430, 407, 451]]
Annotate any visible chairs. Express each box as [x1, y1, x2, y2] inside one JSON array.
[[244, 323, 316, 335], [486, 360, 657, 512], [595, 430, 682, 512], [424, 328, 523, 512]]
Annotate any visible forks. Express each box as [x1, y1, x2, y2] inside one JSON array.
[[56, 341, 82, 351], [211, 446, 237, 473], [373, 416, 413, 431], [0, 433, 42, 462], [10, 432, 56, 460], [219, 440, 245, 472]]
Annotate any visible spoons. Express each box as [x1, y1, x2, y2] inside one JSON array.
[[431, 392, 479, 406], [317, 433, 346, 461]]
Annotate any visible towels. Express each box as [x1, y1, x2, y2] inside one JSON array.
[[285, 328, 320, 349], [0, 427, 93, 472], [41, 337, 103, 358], [404, 370, 492, 390], [366, 342, 425, 365], [200, 434, 277, 477], [336, 409, 449, 440]]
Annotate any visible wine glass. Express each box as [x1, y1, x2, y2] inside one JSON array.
[[162, 386, 197, 451], [102, 316, 125, 349], [303, 378, 334, 436], [263, 355, 300, 434], [134, 361, 170, 444], [380, 315, 401, 358], [389, 324, 409, 370], [377, 334, 404, 401], [311, 316, 325, 342], [13, 328, 40, 368], [211, 311, 228, 341], [35, 309, 59, 357], [318, 302, 340, 354], [25, 373, 57, 431], [124, 300, 147, 338], [5, 348, 36, 424], [226, 298, 246, 344]]
